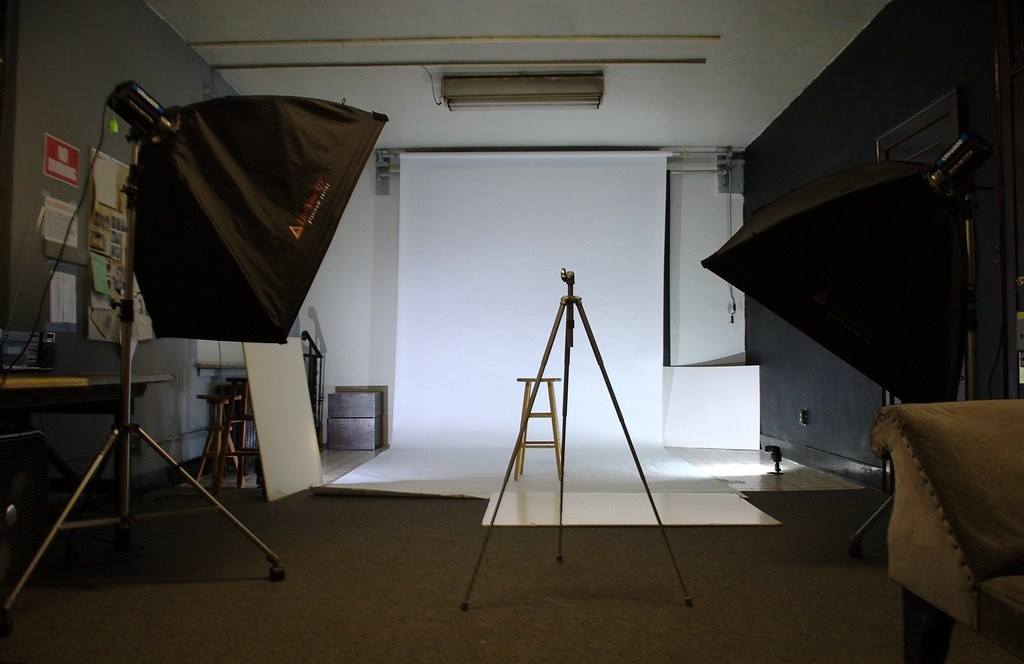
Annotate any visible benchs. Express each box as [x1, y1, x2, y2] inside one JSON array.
[[866, 396, 1024, 664]]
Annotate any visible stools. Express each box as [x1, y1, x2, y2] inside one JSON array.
[[191, 375, 267, 499], [513, 376, 566, 481]]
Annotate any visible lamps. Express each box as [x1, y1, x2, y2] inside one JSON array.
[[440, 72, 607, 113]]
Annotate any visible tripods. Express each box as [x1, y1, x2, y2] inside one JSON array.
[[461, 294, 693, 611]]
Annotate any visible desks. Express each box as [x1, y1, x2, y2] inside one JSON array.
[[0, 368, 178, 550]]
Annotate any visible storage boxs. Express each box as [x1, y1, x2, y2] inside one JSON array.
[[326, 416, 383, 451], [328, 391, 383, 418]]
